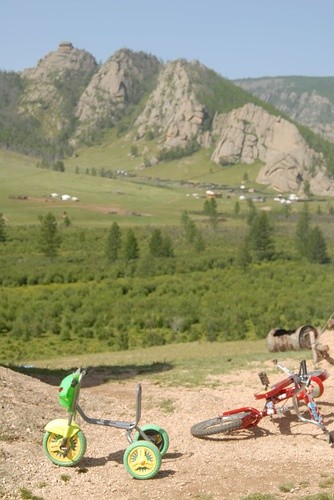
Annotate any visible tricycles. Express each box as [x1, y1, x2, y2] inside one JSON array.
[[41, 369, 169, 480]]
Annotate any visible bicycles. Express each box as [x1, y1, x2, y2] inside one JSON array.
[[189, 358, 334, 446]]
